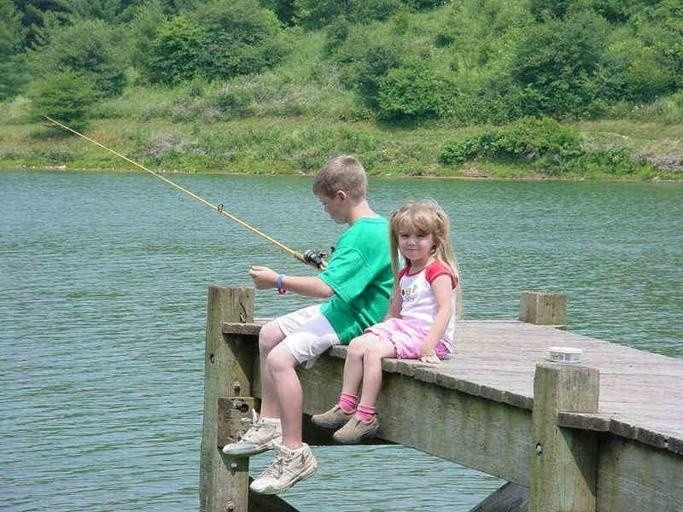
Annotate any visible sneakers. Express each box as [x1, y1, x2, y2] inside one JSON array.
[[221, 407, 286, 457], [248, 436, 320, 497], [310, 401, 357, 429], [332, 412, 381, 444]]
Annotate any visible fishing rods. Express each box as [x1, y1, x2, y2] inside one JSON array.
[[40, 115, 328, 270]]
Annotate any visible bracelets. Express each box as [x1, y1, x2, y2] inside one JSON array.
[[276, 274, 287, 294]]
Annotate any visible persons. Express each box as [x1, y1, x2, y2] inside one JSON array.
[[306, 195, 461, 446], [220, 154, 401, 498]]
